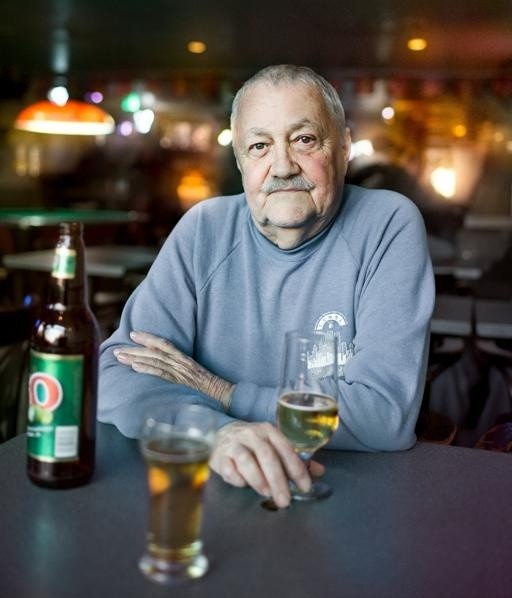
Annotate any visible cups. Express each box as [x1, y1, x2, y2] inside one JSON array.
[[275, 330, 339, 502], [135, 393, 218, 586]]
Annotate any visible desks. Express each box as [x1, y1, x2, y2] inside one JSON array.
[[2, 247, 158, 277], [431, 298, 511, 362]]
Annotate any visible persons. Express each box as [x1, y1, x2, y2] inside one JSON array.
[[97, 67, 435, 508]]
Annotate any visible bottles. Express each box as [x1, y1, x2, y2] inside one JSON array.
[[26, 219, 98, 488]]
